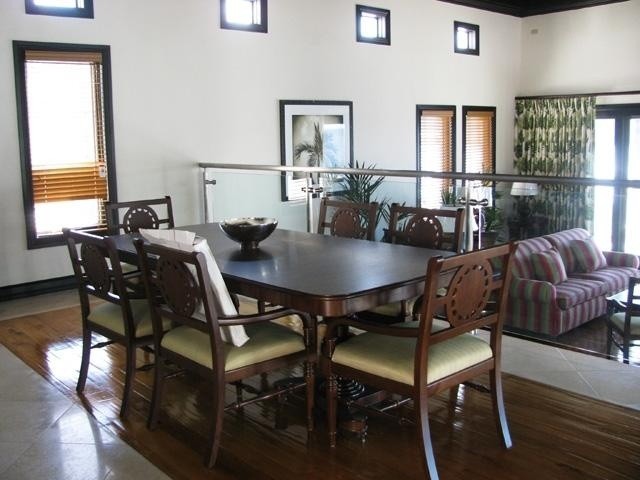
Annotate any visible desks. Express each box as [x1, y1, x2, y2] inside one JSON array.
[[102, 222, 490, 446]]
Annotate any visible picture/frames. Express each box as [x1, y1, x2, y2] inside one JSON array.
[[279, 100, 353, 200]]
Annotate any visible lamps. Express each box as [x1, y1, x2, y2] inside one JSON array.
[[435, 206, 479, 253], [510, 182, 539, 239], [468, 188, 493, 250]]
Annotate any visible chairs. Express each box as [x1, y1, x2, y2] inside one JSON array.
[[104, 196, 175, 298], [605, 277, 640, 364], [62, 227, 192, 419], [322, 240, 519, 480], [317, 197, 378, 241], [338, 203, 464, 341], [132, 238, 317, 468]]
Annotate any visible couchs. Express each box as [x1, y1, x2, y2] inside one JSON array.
[[482, 228, 640, 338]]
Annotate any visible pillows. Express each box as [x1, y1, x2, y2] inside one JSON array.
[[569, 238, 608, 272], [530, 246, 567, 285]]
